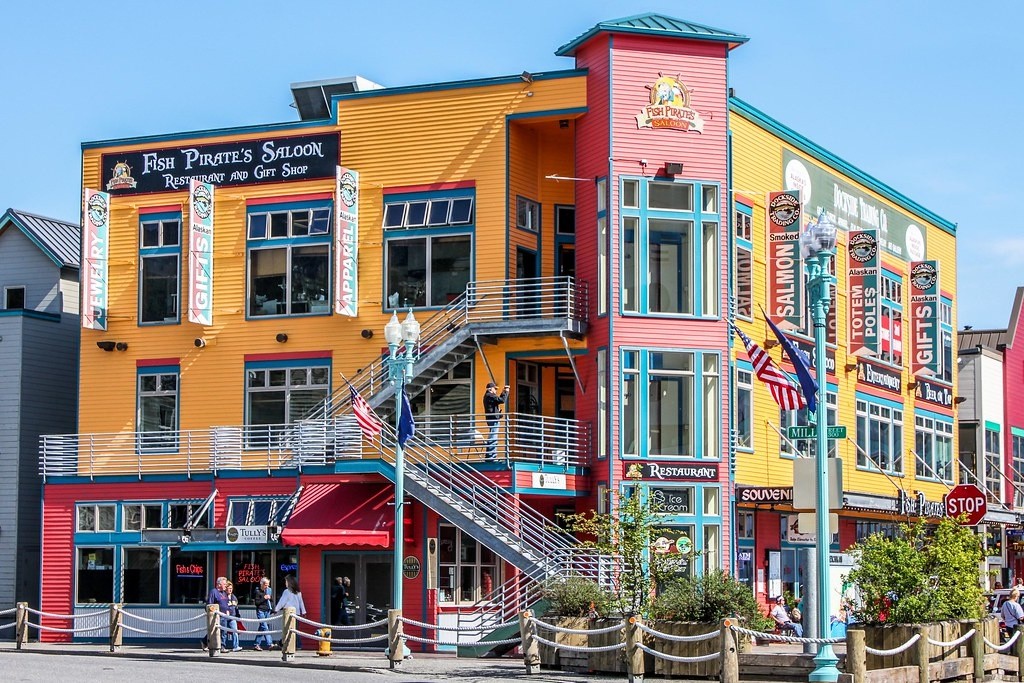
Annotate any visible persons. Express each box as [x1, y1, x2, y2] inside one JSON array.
[[252, 576, 278, 651], [200, 576, 243, 653], [273, 574, 307, 649], [483, 383, 510, 461], [770, 596, 803, 638], [331, 576, 350, 625], [1012, 578, 1024, 590], [995, 582, 1003, 588], [1000, 589, 1024, 656]]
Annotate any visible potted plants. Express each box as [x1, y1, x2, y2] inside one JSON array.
[[842, 512, 1000, 682], [534, 461, 776, 681]]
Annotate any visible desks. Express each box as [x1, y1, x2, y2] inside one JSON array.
[[275, 301, 308, 313]]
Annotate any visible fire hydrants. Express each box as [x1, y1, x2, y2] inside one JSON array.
[[315, 628, 332, 656]]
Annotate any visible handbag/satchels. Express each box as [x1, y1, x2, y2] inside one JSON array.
[[1018, 619, 1024, 624], [227, 620, 246, 635]]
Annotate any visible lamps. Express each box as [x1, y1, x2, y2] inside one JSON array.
[[846, 364, 859, 371], [763, 340, 780, 347], [362, 330, 373, 338], [276, 333, 288, 342], [665, 162, 682, 176], [520, 71, 534, 85], [955, 397, 967, 404], [195, 338, 205, 348], [908, 382, 921, 390], [97, 342, 127, 352]]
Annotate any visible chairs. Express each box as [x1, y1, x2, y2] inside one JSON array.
[[776, 620, 795, 645], [465, 430, 489, 462]]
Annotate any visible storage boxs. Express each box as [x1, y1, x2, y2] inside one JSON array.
[[81, 551, 114, 569]]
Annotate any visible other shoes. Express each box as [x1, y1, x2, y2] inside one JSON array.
[[201, 642, 209, 652], [233, 646, 243, 652], [221, 647, 230, 653]]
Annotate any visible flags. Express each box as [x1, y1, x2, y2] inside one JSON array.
[[350, 386, 383, 443], [734, 326, 803, 410], [763, 311, 820, 417], [398, 379, 414, 452]]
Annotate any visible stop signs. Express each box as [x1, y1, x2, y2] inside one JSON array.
[[945, 484, 987, 526]]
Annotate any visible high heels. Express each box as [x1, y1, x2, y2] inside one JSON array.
[[254, 645, 263, 651], [269, 644, 278, 651]]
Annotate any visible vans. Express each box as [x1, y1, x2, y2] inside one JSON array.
[[986, 589, 1024, 653]]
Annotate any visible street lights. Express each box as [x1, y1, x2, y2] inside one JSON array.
[[799, 210, 841, 683], [385, 308, 420, 660]]
